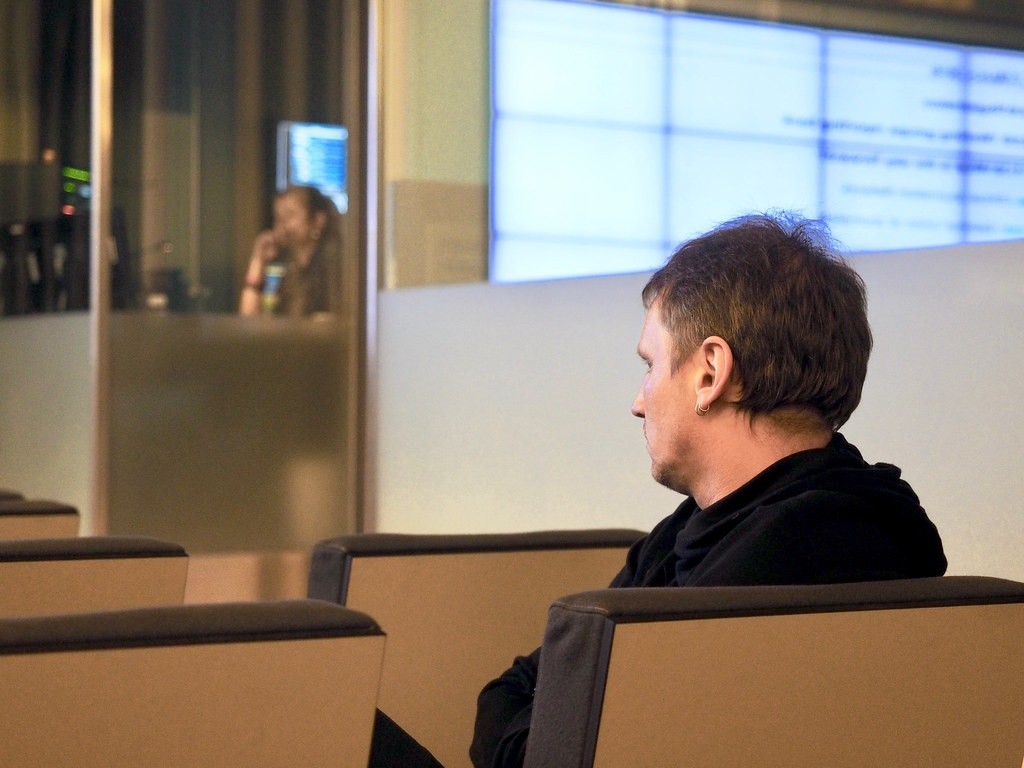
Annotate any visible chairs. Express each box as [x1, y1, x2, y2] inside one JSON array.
[[0, 599, 385, 768], [523, 576, 1024, 768], [307, 529, 650, 768], [0, 535, 189, 619], [0, 501, 81, 541]]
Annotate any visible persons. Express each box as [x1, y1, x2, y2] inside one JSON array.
[[238, 183, 343, 315], [469, 212, 947, 767]]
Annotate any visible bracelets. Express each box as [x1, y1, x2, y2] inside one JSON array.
[[242, 276, 267, 288]]
